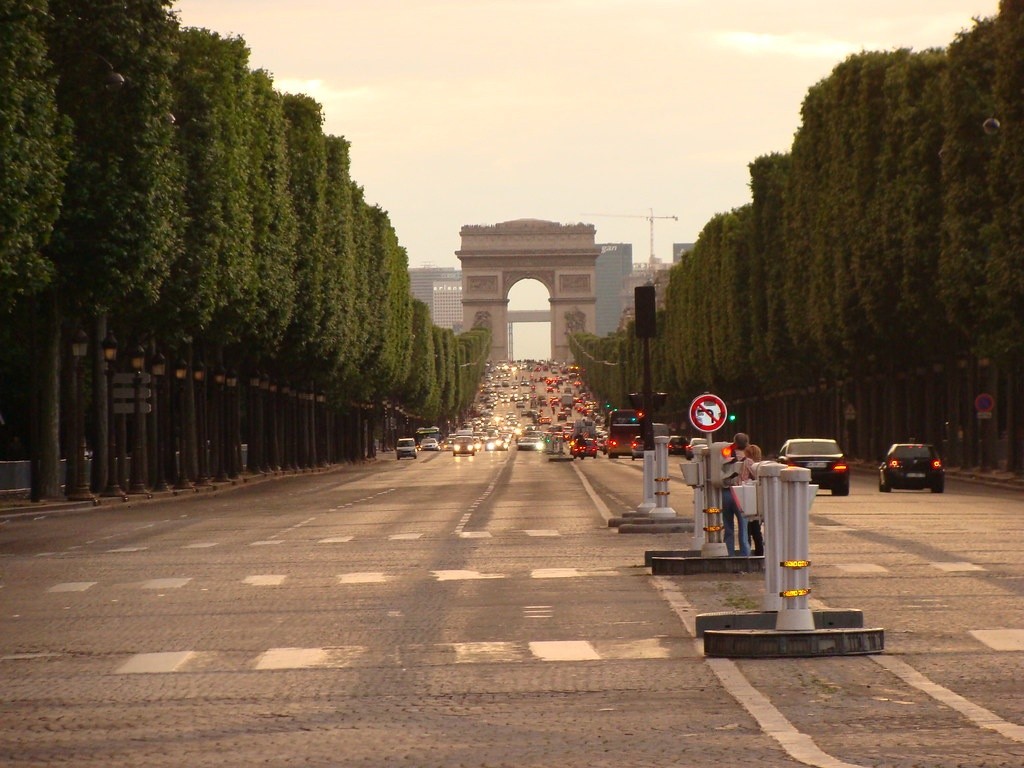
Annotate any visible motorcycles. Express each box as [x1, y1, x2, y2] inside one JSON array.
[[578, 444, 586, 460]]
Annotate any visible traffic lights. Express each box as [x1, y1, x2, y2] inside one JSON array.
[[607, 404, 611, 409], [730, 485, 758, 516], [808, 484, 819, 511], [711, 442, 740, 489], [638, 411, 644, 418], [679, 462, 704, 487], [627, 393, 642, 410], [729, 413, 736, 422], [614, 409, 618, 413], [654, 392, 667, 412]]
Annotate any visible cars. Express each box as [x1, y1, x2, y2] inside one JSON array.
[[879, 443, 945, 492], [775, 439, 849, 496], [632, 436, 646, 460], [453, 436, 475, 456], [573, 438, 598, 459], [445, 358, 619, 456], [667, 436, 688, 456], [397, 438, 418, 460], [420, 438, 439, 451], [685, 437, 709, 460]]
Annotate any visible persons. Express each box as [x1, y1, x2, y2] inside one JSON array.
[[744, 444, 765, 556], [721, 433, 756, 556]]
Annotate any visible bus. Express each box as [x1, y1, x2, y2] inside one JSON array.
[[416, 426, 439, 445], [607, 409, 641, 458]]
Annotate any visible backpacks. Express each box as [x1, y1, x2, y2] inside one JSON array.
[[718, 451, 746, 489]]
